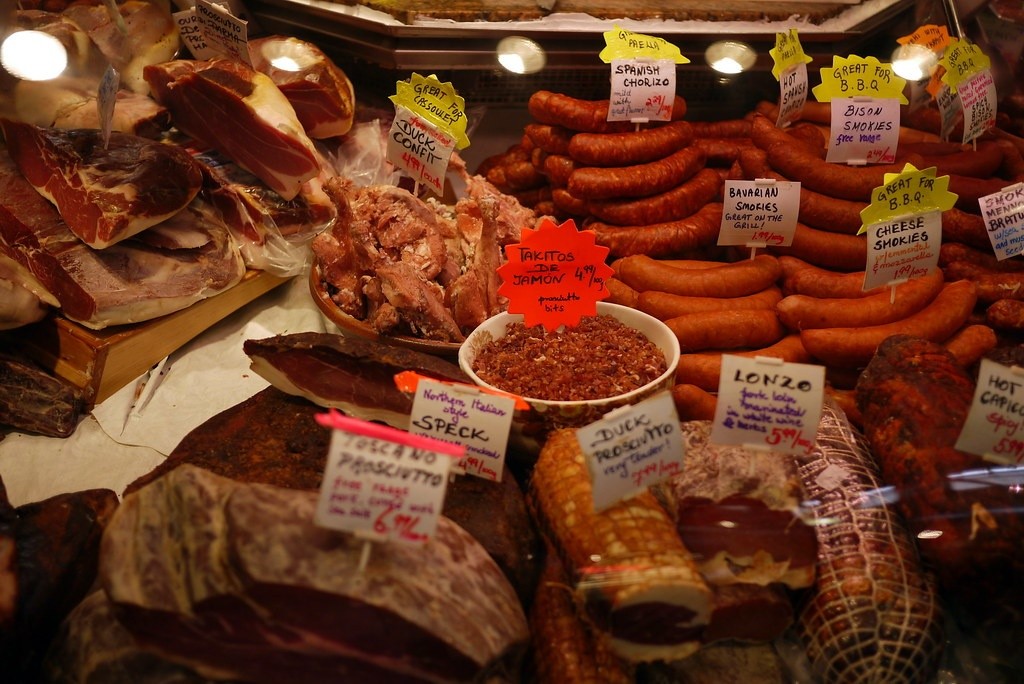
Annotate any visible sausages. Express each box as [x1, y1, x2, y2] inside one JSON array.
[[485, 86, 1023, 418]]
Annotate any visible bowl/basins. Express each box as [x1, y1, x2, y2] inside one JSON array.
[[458, 299, 681, 424], [308, 256, 465, 359]]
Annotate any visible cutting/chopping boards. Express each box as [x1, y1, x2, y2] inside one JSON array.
[[4, 264, 301, 412]]
